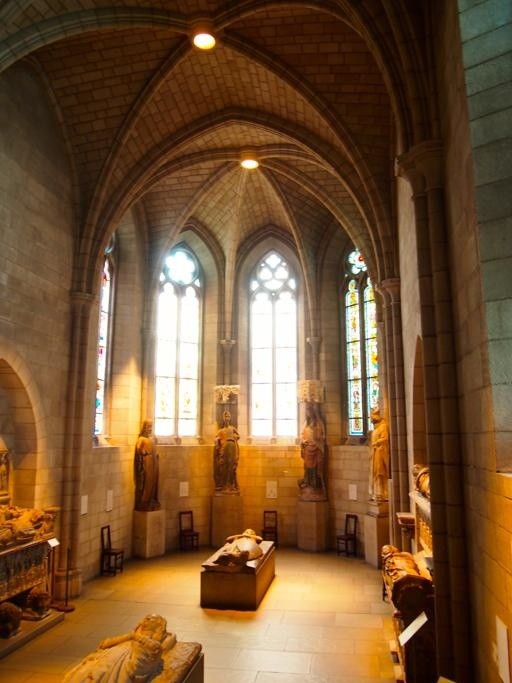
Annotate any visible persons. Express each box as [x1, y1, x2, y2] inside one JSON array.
[[380, 544, 421, 597], [136, 418, 162, 511], [214, 527, 263, 565], [214, 409, 239, 492], [63, 612, 167, 683], [369, 408, 391, 502], [0, 503, 42, 548], [299, 403, 327, 494], [412, 463, 430, 499]]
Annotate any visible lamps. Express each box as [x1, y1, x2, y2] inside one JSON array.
[[189, 11, 216, 51], [241, 151, 259, 170]]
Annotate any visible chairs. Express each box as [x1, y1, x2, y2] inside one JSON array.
[[179, 511, 199, 551], [261, 510, 278, 549], [337, 514, 357, 558], [99, 525, 124, 576]]
